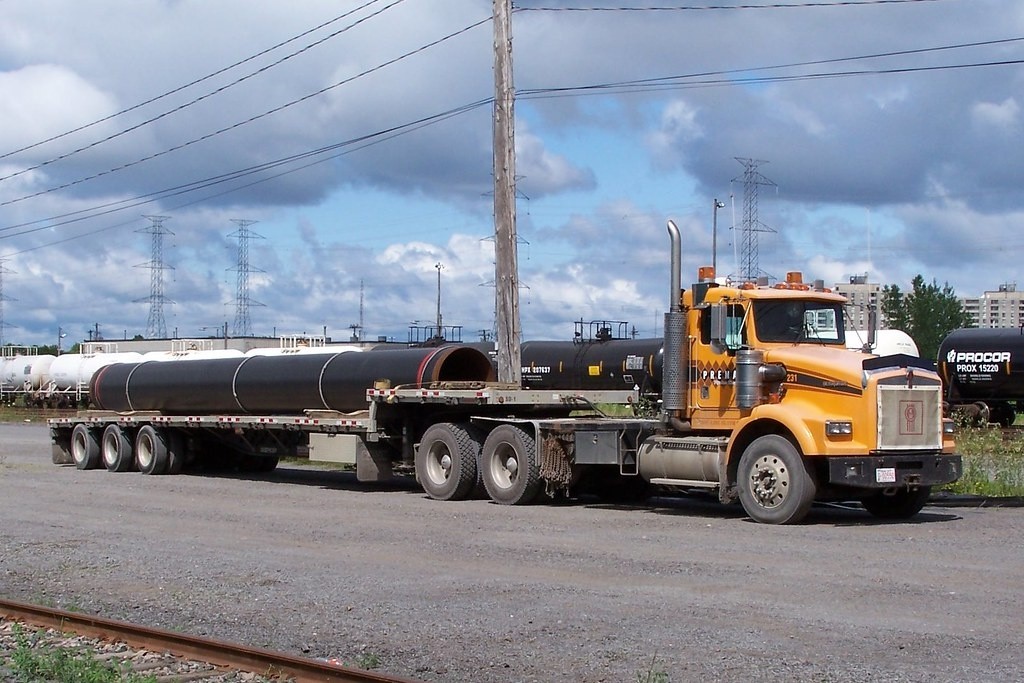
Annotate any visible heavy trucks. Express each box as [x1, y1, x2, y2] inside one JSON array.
[[46, 218, 964, 525], [937, 324, 1024, 429]]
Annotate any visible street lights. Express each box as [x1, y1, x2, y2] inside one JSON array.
[[713, 199, 726, 279], [435, 262, 445, 326]]
